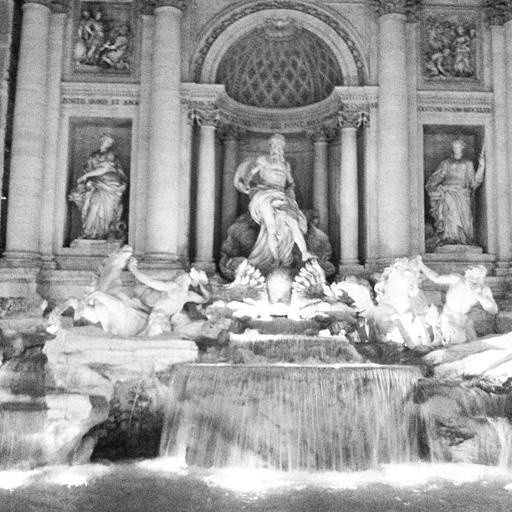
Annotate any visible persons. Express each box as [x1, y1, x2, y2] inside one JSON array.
[[423, 20, 477, 78], [77, 7, 130, 71]]
